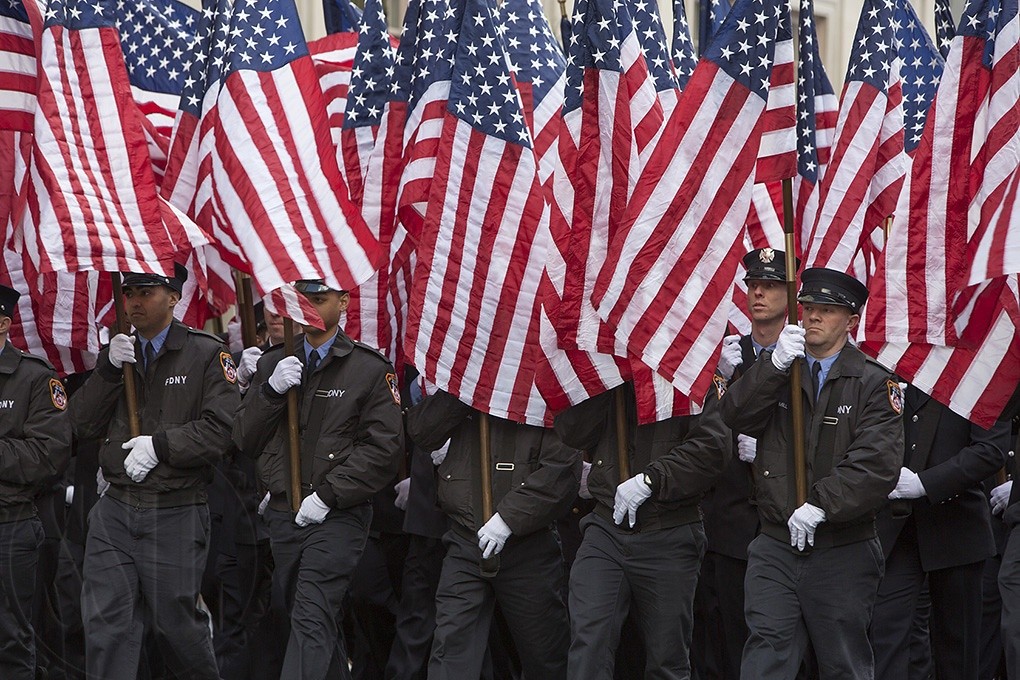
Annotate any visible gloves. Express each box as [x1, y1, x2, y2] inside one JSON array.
[[236, 347, 262, 385], [121, 435, 160, 483], [738, 434, 757, 462], [888, 467, 927, 498], [771, 324, 807, 371], [295, 492, 330, 527], [613, 472, 653, 528], [788, 502, 827, 551], [268, 355, 304, 394], [716, 333, 743, 380], [108, 334, 138, 369], [478, 512, 512, 558], [579, 461, 594, 499], [431, 438, 450, 466], [394, 478, 412, 510], [990, 480, 1013, 518]]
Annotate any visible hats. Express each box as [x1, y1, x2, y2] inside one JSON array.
[[294, 278, 349, 294], [0, 284, 21, 318], [797, 267, 868, 313], [120, 260, 188, 300], [742, 247, 801, 282]]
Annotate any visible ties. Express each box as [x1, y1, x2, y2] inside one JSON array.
[[306, 350, 320, 381], [812, 360, 821, 404]]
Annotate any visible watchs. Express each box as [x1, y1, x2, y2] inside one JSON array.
[[643, 473, 654, 491]]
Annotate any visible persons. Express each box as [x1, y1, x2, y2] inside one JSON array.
[[554, 379, 732, 680], [404, 388, 581, 680], [691, 246, 1020, 680], [66, 261, 301, 680], [0, 285, 108, 680], [232, 279, 471, 680]]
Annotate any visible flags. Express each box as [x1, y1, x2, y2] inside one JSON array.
[[0, 0, 1020, 432]]
